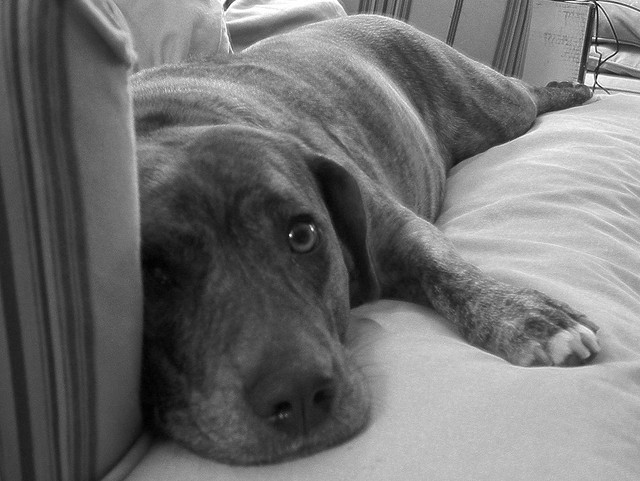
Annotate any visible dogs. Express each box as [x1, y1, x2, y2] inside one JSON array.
[[131, 14, 601, 466]]
